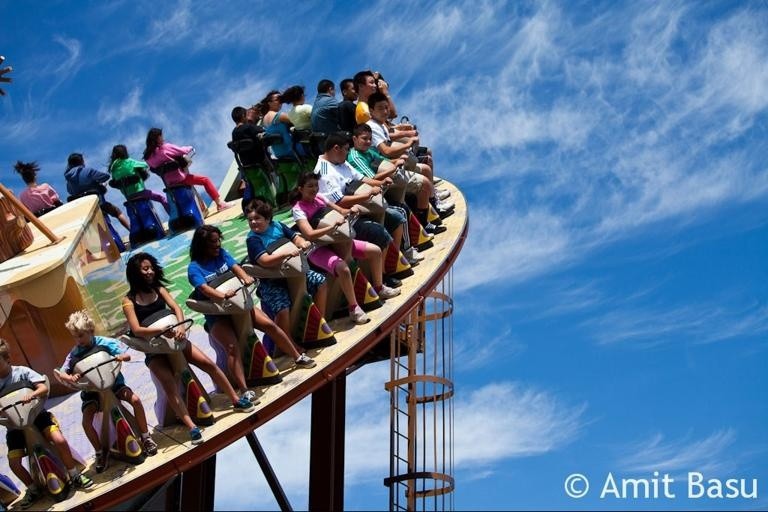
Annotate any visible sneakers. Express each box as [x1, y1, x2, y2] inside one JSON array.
[[295, 352, 316, 368], [348, 179, 455, 325], [217, 201, 235, 212], [189, 427, 204, 444], [70, 473, 97, 491], [233, 397, 254, 413], [240, 390, 260, 406], [20, 485, 42, 509], [95, 446, 110, 473], [141, 432, 158, 456]]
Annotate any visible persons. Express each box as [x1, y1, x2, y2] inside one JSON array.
[[187, 225, 317, 407], [0, 339, 98, 511], [107, 144, 170, 217], [141, 127, 235, 212], [59, 307, 159, 475], [13, 161, 60, 222], [233, 68, 457, 355], [64, 153, 131, 231], [121, 252, 257, 445]]
